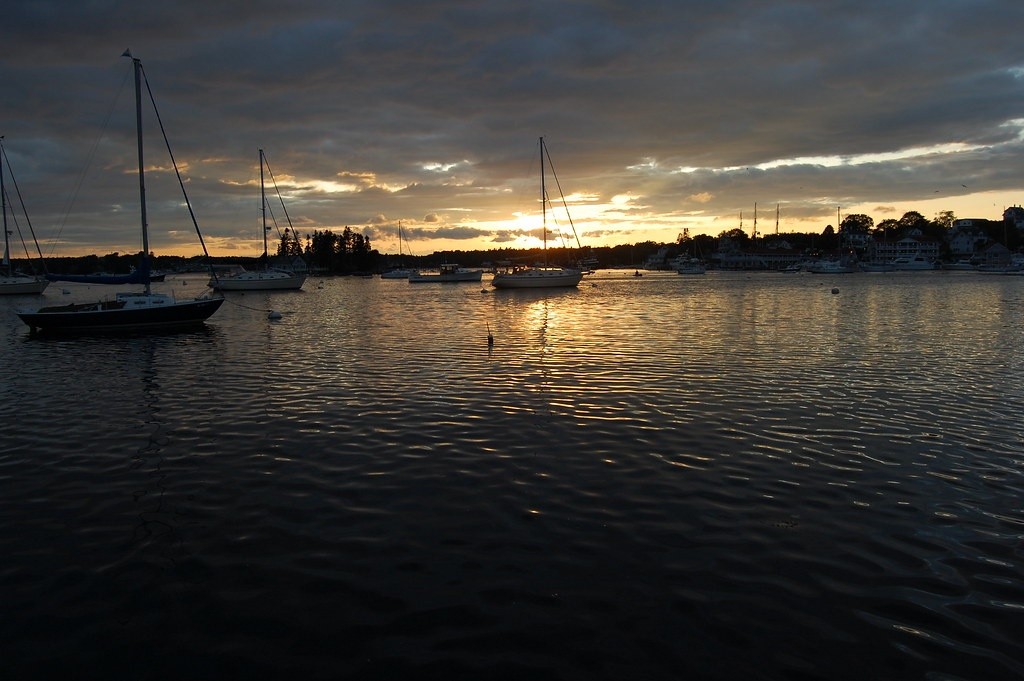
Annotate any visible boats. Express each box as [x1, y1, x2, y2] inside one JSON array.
[[780, 245, 1024, 274], [351, 269, 373, 278], [42, 268, 141, 285], [151, 271, 170, 283], [404, 268, 483, 282]]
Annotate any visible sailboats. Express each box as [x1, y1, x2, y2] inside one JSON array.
[[18, 45, 228, 334], [379, 221, 420, 279], [206, 145, 310, 291], [487, 135, 589, 287], [0, 136, 52, 300], [675, 234, 708, 276]]
